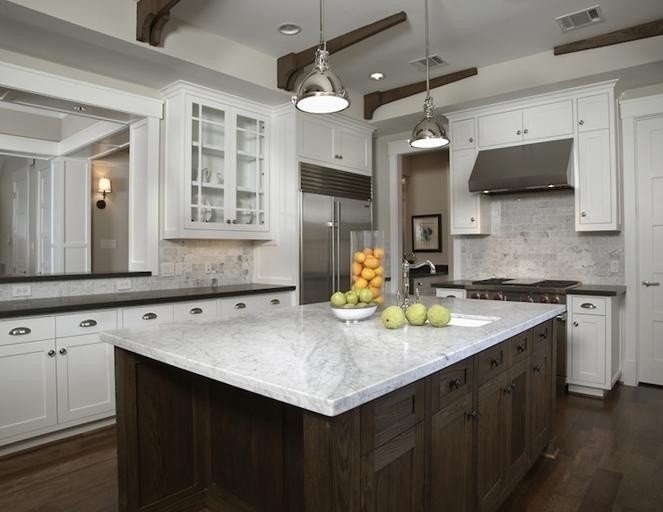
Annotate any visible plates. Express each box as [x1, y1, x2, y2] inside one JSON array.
[[237, 198, 253, 224], [191, 195, 211, 223]]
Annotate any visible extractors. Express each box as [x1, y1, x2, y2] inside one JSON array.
[[466, 137, 575, 196]]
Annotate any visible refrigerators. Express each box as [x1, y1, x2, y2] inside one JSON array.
[[297, 160, 373, 307]]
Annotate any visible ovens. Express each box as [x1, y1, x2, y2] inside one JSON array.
[[557, 311, 567, 394]]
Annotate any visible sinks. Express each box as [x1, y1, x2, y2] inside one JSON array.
[[425, 313, 501, 329]]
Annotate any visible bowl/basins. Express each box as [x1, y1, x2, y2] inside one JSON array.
[[329, 301, 377, 323]]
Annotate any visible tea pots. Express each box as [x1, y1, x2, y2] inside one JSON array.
[[209, 172, 223, 185]]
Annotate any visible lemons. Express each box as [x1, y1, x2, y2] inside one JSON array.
[[351, 248, 384, 305]]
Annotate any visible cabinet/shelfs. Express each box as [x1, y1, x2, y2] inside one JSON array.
[[164, 80, 273, 238], [574, 76, 625, 235], [213, 292, 292, 317], [476, 90, 574, 153], [434, 288, 464, 299], [446, 108, 491, 238], [566, 297, 624, 394], [114, 334, 428, 511], [0, 307, 119, 447], [529, 316, 565, 458], [277, 102, 372, 175], [426, 357, 476, 510], [122, 298, 215, 325], [472, 330, 530, 502]]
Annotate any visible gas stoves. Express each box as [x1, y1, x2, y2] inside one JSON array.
[[466, 274, 580, 305]]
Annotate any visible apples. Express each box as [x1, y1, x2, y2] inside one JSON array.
[[330, 288, 373, 306], [405, 304, 428, 326], [381, 305, 405, 329], [428, 304, 450, 327]]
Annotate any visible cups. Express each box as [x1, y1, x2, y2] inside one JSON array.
[[194, 166, 210, 182], [348, 228, 385, 312]]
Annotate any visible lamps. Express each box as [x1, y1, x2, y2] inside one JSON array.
[[293, 1, 352, 116], [405, 2, 450, 154], [93, 174, 113, 211]]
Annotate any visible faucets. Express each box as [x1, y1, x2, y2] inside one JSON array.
[[402, 259, 436, 302]]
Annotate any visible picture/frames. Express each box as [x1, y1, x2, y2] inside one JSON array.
[[410, 213, 443, 253]]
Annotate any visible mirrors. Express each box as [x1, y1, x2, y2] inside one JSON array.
[[0, 86, 130, 283]]
